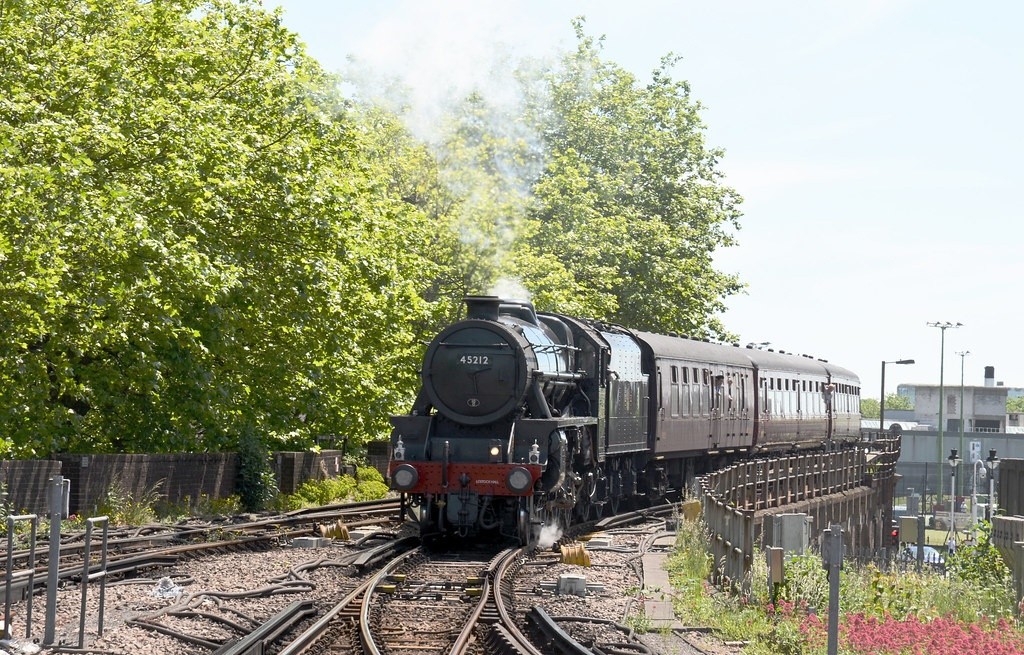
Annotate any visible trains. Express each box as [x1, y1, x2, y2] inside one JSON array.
[[385, 295, 867, 552]]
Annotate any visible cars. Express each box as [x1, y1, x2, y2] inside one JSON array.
[[901, 546, 946, 576]]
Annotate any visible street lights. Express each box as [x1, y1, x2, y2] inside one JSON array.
[[971, 459, 988, 563], [925, 320, 965, 512], [987, 447, 1000, 534], [879, 359, 916, 433], [954, 350, 973, 509], [942, 448, 961, 547]]
